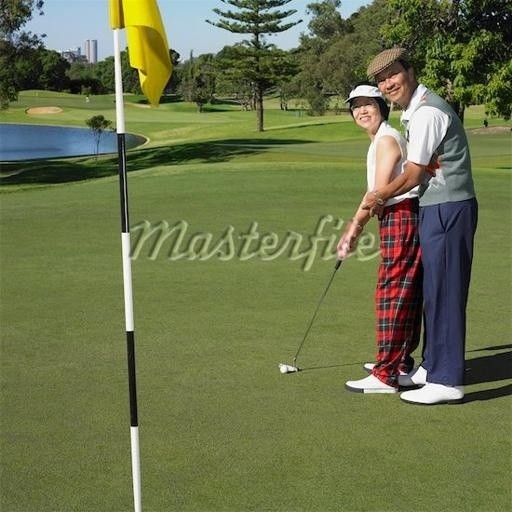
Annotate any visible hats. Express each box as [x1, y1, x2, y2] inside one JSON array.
[[343, 85, 386, 102], [367, 48, 409, 81]]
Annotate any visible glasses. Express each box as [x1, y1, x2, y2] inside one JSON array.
[[350, 101, 374, 111]]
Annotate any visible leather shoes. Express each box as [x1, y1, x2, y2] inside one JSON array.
[[399, 380, 464, 404], [397, 364, 428, 388]]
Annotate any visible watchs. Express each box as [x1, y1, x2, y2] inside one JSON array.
[[373, 189, 387, 208]]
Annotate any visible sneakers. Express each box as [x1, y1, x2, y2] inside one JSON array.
[[344, 373, 397, 394], [363, 362, 376, 372]]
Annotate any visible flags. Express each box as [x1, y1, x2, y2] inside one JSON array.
[[109, 0, 172, 112]]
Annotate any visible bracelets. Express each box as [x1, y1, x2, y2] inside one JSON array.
[[352, 217, 364, 232]]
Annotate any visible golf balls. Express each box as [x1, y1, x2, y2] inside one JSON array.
[[281, 366, 287, 372]]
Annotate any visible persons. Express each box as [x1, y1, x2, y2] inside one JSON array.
[[360, 47, 479, 405], [336, 82, 422, 395]]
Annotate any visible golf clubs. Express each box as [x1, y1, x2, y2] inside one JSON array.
[[279, 256, 343, 372]]
